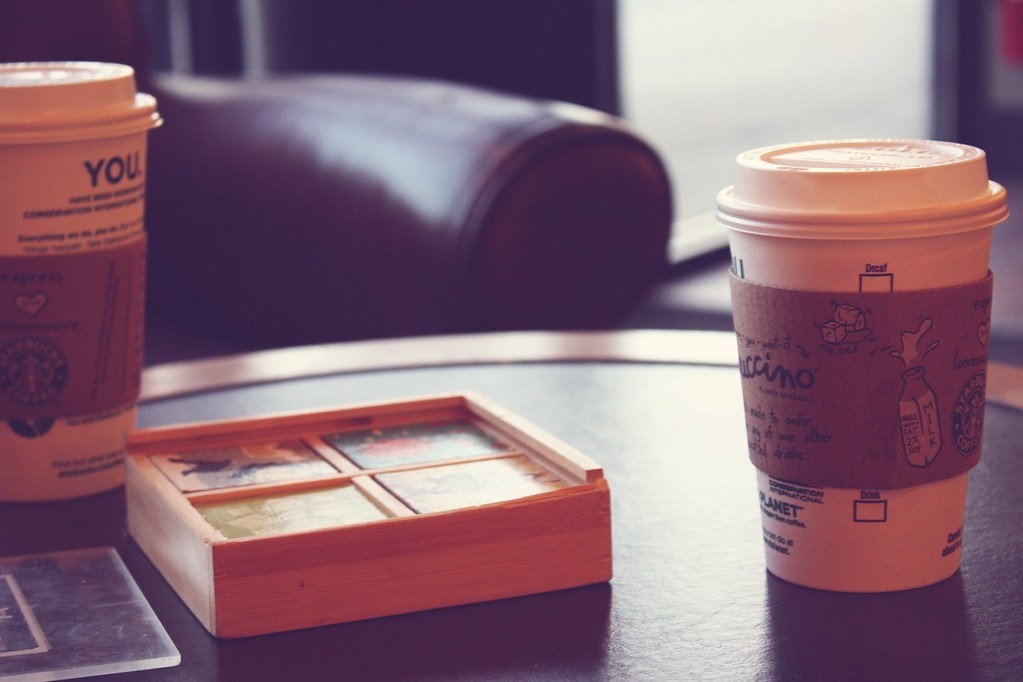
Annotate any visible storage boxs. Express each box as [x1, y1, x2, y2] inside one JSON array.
[[125, 392, 615, 645]]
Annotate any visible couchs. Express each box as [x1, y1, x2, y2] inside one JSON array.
[[0, 0, 675, 365]]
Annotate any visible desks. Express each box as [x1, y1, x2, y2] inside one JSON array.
[[0, 332, 1023, 682]]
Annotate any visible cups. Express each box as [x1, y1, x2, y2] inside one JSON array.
[[0, 58, 163, 503], [714, 138, 1007, 593]]
